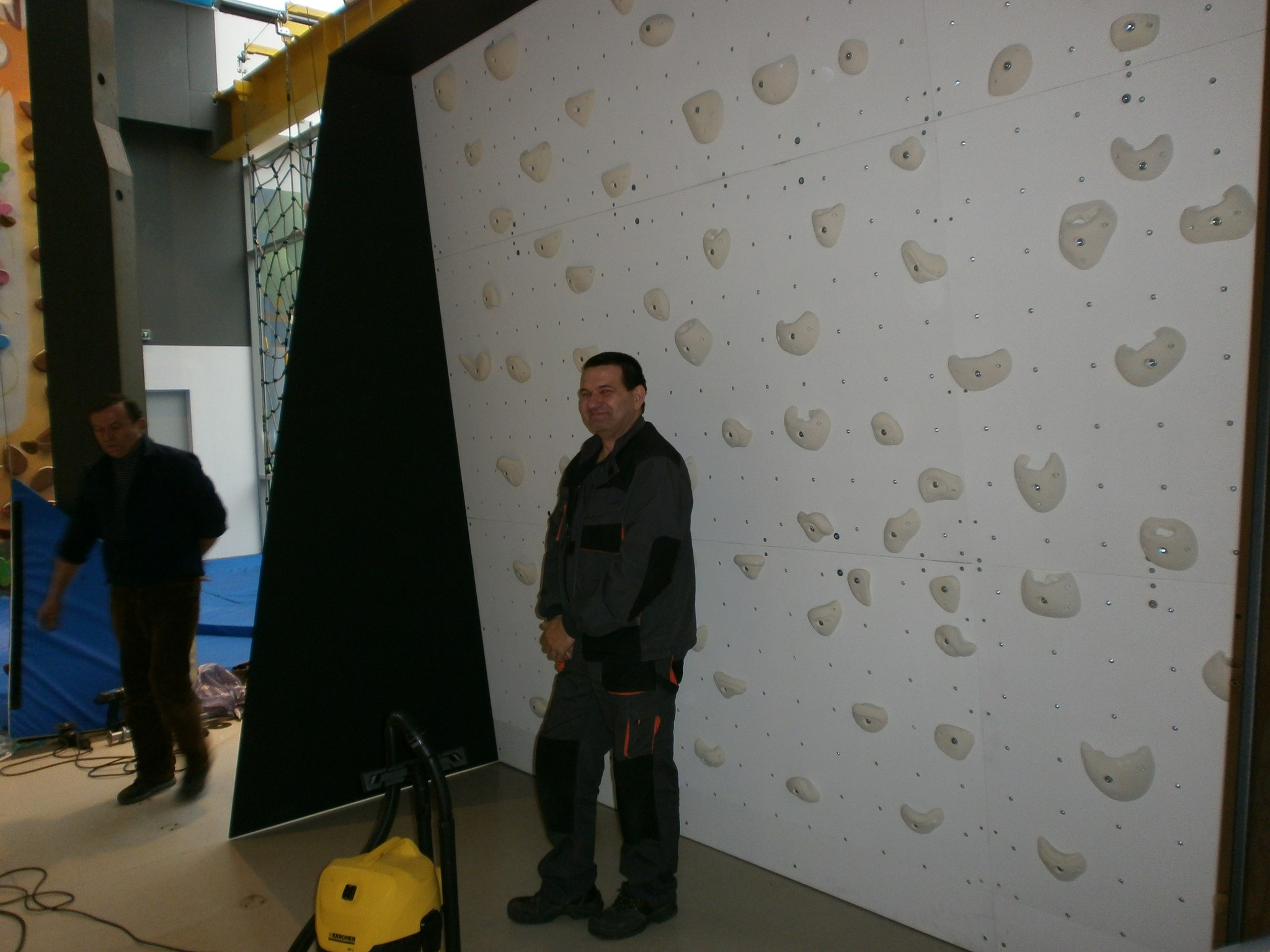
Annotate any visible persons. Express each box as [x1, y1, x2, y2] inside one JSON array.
[[505, 350, 696, 938], [39, 393, 232, 803]]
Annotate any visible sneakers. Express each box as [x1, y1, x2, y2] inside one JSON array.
[[588, 882, 677, 939], [507, 880, 603, 924]]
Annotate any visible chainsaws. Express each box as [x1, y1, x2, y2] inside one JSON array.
[[52, 722, 80, 745]]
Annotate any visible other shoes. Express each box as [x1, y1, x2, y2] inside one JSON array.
[[180, 757, 212, 805], [117, 770, 177, 805]]
